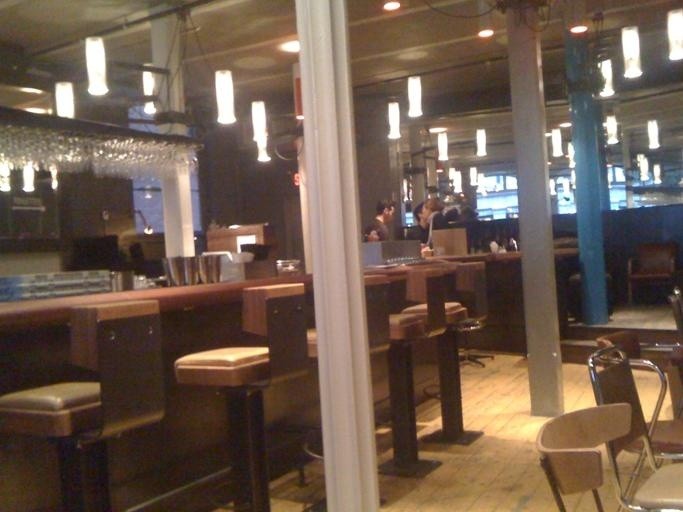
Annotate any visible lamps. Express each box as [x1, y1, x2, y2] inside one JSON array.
[[1, 6, 682, 195]]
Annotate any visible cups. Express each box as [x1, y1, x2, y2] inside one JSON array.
[[383, 255, 421, 267], [276, 259, 301, 278], [107, 254, 222, 293]]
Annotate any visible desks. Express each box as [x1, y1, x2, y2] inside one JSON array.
[[442, 245, 580, 333]]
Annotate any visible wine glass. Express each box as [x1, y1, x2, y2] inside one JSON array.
[[0, 122, 200, 181]]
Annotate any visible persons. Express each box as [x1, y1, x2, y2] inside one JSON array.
[[402, 199, 432, 244], [364, 196, 397, 243]]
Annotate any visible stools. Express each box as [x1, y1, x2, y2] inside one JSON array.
[[295, 275, 391, 493], [2, 298, 167, 509], [173, 282, 305, 512], [375, 266, 447, 482], [425, 261, 490, 446]]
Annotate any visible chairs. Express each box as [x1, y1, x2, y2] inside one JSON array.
[[624, 239, 681, 303], [594, 327, 682, 462], [531, 401, 633, 510], [587, 344, 681, 511]]
